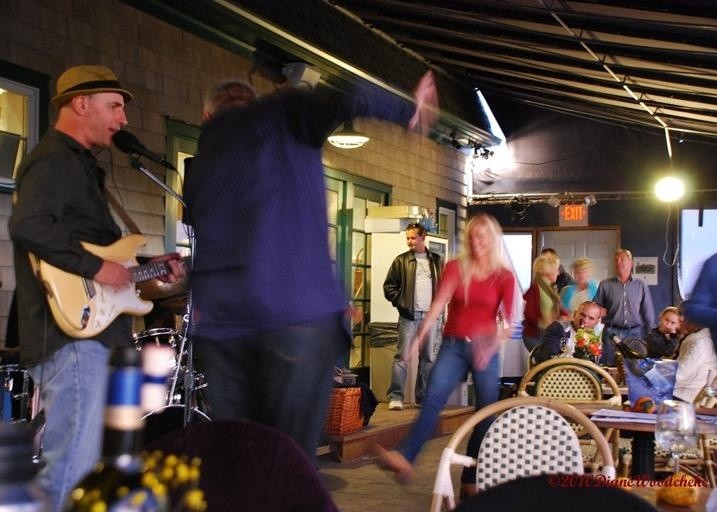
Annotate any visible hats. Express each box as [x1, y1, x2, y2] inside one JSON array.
[[50, 63, 134, 109]]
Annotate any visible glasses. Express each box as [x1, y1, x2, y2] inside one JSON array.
[[408, 223, 420, 228]]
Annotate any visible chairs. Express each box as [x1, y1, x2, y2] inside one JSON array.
[[429, 396, 617, 512], [518, 357, 622, 406]]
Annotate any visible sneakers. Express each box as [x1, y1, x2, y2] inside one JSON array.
[[389, 400, 403, 411]]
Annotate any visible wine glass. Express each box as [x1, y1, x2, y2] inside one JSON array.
[[654, 399, 695, 475], [561, 338, 574, 357]]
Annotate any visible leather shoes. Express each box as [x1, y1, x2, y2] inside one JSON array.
[[375, 444, 412, 485], [460, 483, 474, 498]]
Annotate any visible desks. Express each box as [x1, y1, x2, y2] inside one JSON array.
[[557, 396, 717, 489]]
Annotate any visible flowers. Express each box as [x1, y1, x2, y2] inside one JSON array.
[[574, 328, 603, 366]]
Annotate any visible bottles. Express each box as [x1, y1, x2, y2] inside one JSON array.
[[609, 333, 675, 399]]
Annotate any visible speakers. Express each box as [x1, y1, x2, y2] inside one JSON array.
[[281, 63, 321, 89]]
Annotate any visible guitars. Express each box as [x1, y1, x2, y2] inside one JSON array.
[[28, 234, 192, 339]]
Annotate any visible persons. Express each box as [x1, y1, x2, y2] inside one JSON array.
[[178, 66, 433, 468], [646, 307, 682, 359], [560, 258, 600, 319], [522, 257, 568, 355], [672, 302, 717, 408], [541, 248, 575, 291], [686, 253, 717, 353], [372, 215, 516, 486], [533, 300, 600, 378], [5, 65, 182, 512], [593, 249, 656, 367], [383, 223, 444, 410]]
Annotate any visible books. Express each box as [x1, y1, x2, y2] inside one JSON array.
[[590, 408, 658, 425]]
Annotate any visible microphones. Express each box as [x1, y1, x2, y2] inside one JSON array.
[[109, 130, 177, 177]]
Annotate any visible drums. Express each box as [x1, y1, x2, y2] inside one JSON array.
[[134, 328, 180, 347], [0, 365, 34, 423]]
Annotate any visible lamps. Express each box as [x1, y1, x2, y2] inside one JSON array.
[[584, 194, 598, 207], [465, 137, 496, 159], [547, 194, 564, 208], [327, 112, 370, 150], [654, 171, 686, 204]]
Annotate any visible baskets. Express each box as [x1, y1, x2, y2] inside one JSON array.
[[321, 387, 366, 438]]
